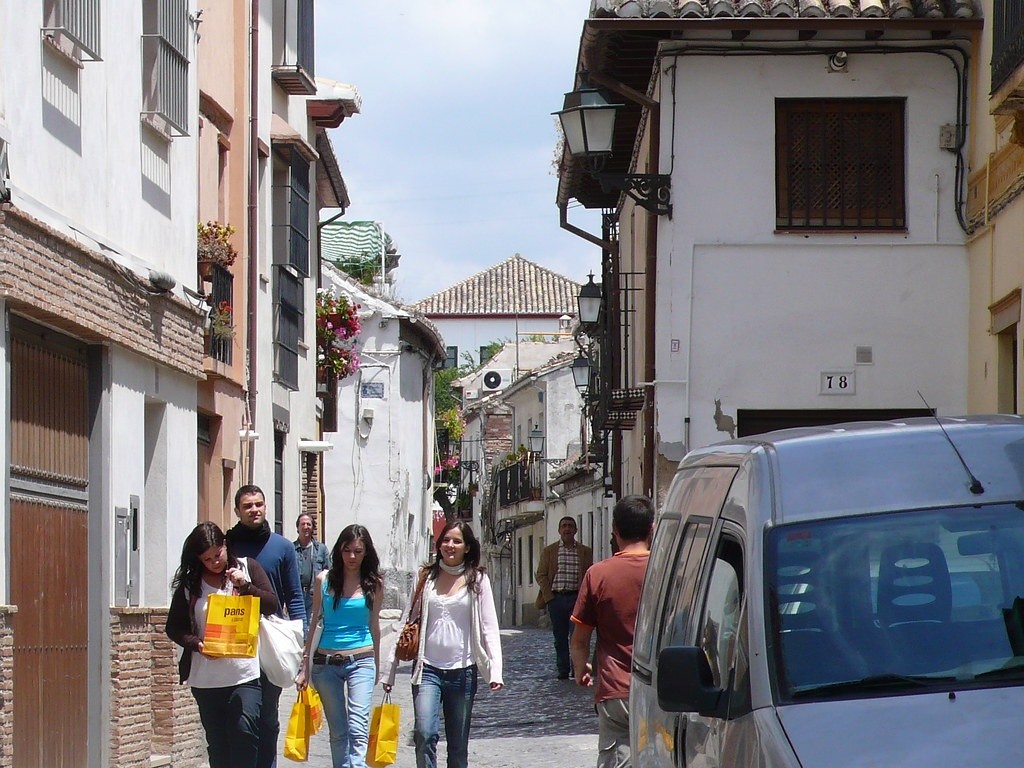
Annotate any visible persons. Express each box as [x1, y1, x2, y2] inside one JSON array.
[[536, 515, 594, 680], [166, 522, 279, 768], [294, 525, 384, 768], [291, 513, 331, 631], [570, 495, 657, 768], [223, 485, 308, 768], [655, 604, 721, 767], [380, 520, 504, 768]]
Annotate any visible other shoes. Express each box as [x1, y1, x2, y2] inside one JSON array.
[[558, 674, 569, 679]]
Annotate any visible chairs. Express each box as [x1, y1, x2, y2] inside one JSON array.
[[876, 543, 955, 673], [776, 552, 860, 686]]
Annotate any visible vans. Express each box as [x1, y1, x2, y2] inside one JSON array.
[[629, 412, 1024, 768]]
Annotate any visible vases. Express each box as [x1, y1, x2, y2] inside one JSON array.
[[316, 313, 342, 345], [316, 362, 345, 384], [204, 329, 212, 355], [528, 487, 542, 500], [434, 469, 448, 483], [197, 256, 229, 282], [435, 419, 450, 430]]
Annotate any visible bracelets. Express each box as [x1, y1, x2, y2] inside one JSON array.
[[303, 654, 310, 658]]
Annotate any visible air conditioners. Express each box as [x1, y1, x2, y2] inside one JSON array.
[[482, 369, 511, 392]]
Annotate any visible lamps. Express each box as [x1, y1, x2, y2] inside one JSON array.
[[574, 270, 607, 346], [568, 349, 601, 402], [559, 314, 572, 333], [363, 408, 374, 420], [527, 421, 566, 469], [378, 319, 389, 328], [549, 62, 673, 221], [829, 50, 849, 71]]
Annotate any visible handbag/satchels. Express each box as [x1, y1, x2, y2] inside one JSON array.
[[283, 702, 311, 762], [299, 684, 324, 734], [395, 571, 429, 661], [203, 594, 261, 658], [258, 612, 304, 689], [366, 690, 400, 767]]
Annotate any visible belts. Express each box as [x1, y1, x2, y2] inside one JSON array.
[[302, 587, 313, 591], [313, 651, 375, 666]]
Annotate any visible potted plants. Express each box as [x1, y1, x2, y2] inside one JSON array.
[[506, 444, 526, 464], [467, 481, 479, 496], [455, 489, 471, 518]]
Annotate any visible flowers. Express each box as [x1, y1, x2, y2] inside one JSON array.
[[197, 220, 239, 267], [435, 451, 464, 486], [316, 284, 362, 376], [209, 300, 237, 341], [438, 405, 466, 443]]
